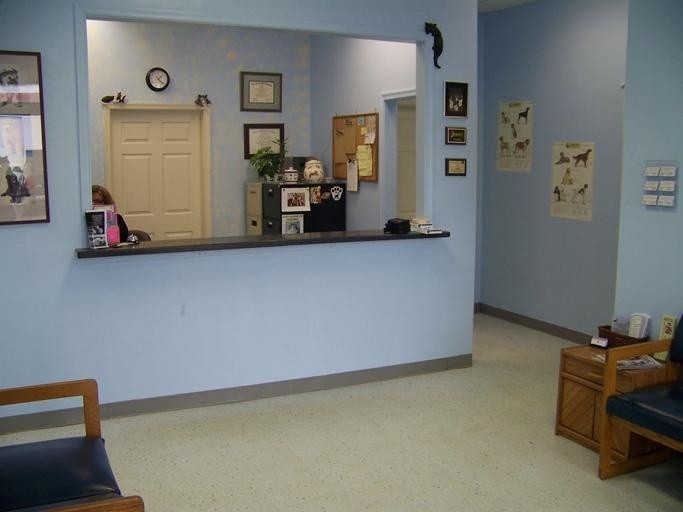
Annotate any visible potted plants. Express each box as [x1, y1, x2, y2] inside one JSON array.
[[247, 136, 289, 183]]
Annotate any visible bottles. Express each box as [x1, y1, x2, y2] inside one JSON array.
[[303, 159, 324, 185]]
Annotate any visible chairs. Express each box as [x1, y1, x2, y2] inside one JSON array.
[[127, 230, 151, 242], [0, 379, 144, 512], [598, 311, 683, 480]]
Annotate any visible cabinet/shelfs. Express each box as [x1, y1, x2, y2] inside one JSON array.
[[246, 183, 346, 236], [555, 341, 670, 464]]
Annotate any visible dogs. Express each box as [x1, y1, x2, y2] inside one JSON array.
[[0, 68, 23, 109]]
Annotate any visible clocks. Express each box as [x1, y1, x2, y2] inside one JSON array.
[[146, 68, 169, 91]]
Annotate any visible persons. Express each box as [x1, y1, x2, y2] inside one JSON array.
[[92, 185, 129, 242]]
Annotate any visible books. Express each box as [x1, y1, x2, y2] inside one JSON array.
[[594, 352, 664, 371], [85, 204, 118, 248]]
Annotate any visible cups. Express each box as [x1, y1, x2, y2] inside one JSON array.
[[284, 166, 298, 184]]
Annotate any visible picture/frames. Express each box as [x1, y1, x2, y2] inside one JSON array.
[[445, 126, 467, 145], [243, 124, 285, 160], [443, 80, 468, 119], [0, 50, 50, 225], [240, 71, 283, 112], [446, 158, 467, 175]]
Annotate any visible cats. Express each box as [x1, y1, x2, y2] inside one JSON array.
[[424, 22, 443, 69]]
